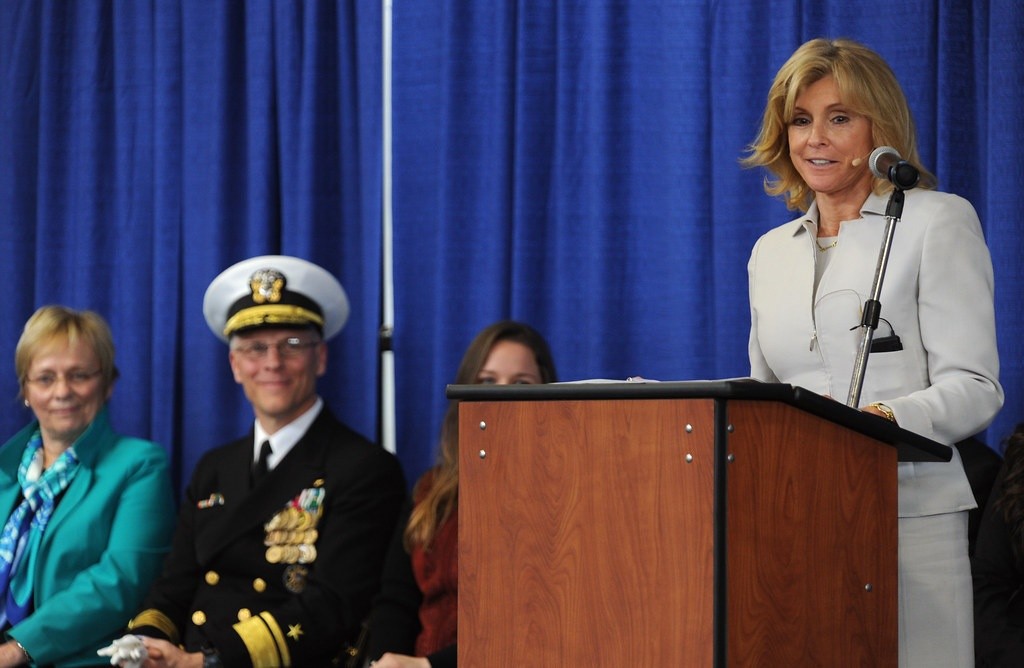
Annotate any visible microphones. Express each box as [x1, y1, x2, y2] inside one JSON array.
[[852, 147, 874, 167], [868, 146, 919, 191]]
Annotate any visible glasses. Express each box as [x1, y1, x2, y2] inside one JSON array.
[[229, 336, 319, 357], [25, 369, 102, 385]]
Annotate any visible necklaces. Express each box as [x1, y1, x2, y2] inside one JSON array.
[[816, 216, 861, 252]]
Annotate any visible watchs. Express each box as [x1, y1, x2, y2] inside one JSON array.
[[868, 402, 894, 422]]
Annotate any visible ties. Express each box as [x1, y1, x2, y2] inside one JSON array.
[[250, 441, 272, 490]]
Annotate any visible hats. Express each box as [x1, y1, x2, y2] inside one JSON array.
[[202, 255, 349, 342]]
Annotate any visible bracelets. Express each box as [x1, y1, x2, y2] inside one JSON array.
[[18, 643, 31, 661]]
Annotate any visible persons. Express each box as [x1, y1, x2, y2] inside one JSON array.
[[741, 37, 1004, 668], [373, 322, 557, 668], [0, 307, 176, 668], [99, 256, 407, 668]]
